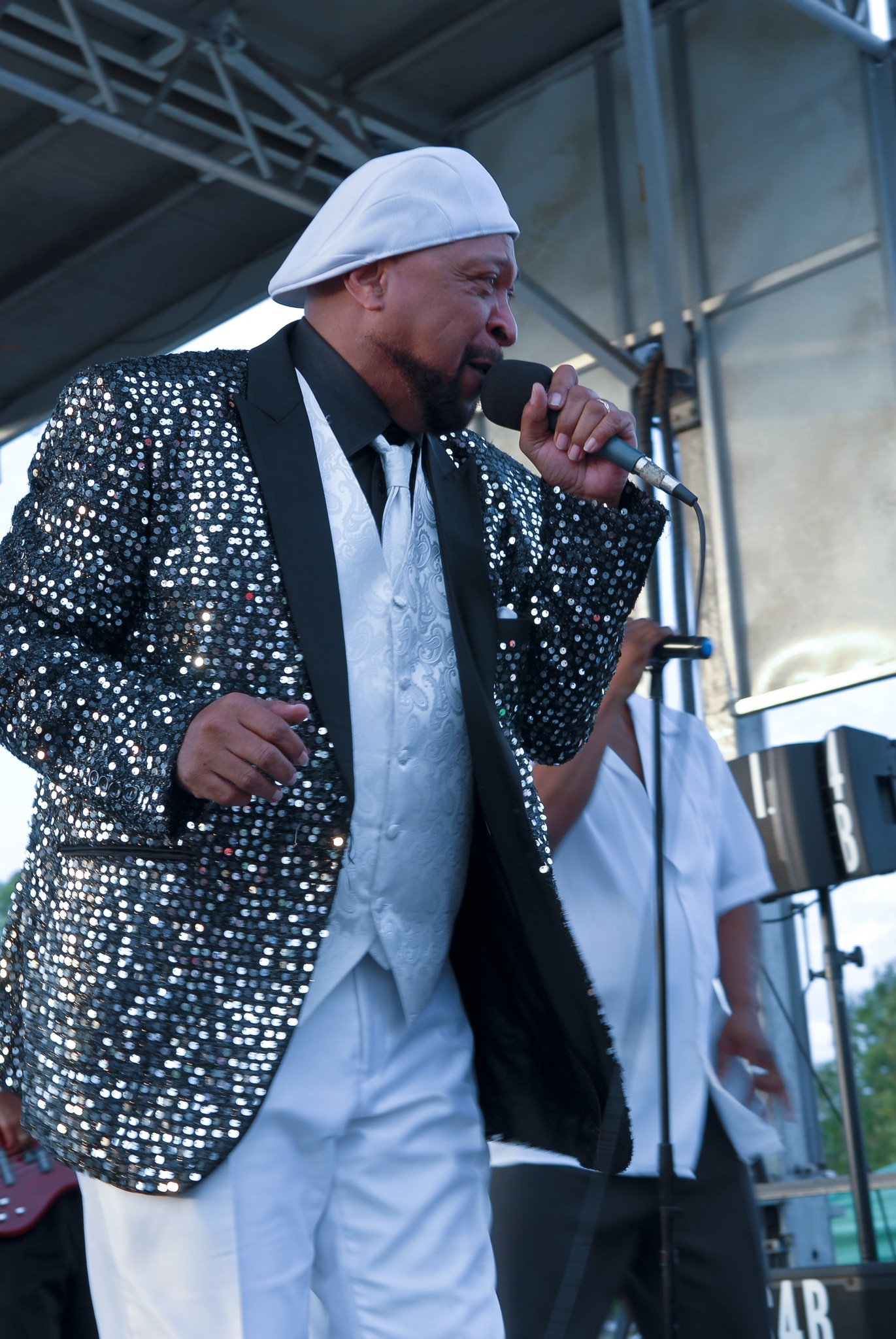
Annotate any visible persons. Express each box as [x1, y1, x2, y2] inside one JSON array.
[[0, 872, 100, 1339], [487, 618, 794, 1339], [0, 146, 668, 1339]]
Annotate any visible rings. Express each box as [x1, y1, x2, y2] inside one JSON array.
[[18, 1133, 26, 1141], [597, 398, 610, 413]]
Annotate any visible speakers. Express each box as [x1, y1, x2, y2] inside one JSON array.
[[725, 723, 896, 899]]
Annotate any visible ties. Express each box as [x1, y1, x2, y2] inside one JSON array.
[[375, 432, 419, 588]]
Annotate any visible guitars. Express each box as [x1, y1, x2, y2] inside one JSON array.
[[0, 1084, 79, 1237]]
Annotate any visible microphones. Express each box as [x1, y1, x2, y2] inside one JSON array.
[[480, 359, 699, 507], [652, 635, 713, 660]]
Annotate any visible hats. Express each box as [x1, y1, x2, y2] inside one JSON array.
[[267, 148, 521, 307]]
